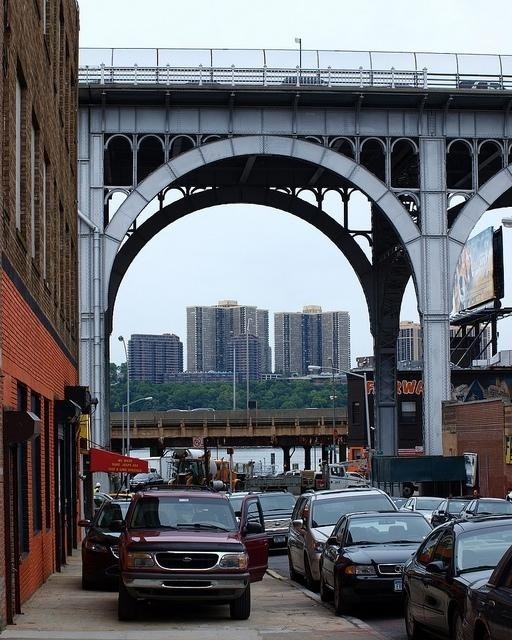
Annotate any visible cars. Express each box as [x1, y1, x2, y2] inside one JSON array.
[[166, 408, 216, 412]]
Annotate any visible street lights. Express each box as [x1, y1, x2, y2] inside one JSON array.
[[118, 335, 153, 493], [230, 318, 253, 411], [307, 357, 372, 450]]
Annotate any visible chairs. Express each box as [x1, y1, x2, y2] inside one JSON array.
[[388, 526, 405, 537], [193, 512, 217, 522], [139, 511, 168, 525]]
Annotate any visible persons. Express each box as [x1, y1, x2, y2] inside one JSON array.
[[94, 482, 103, 507], [505, 488, 512, 504], [472, 488, 480, 496]]
